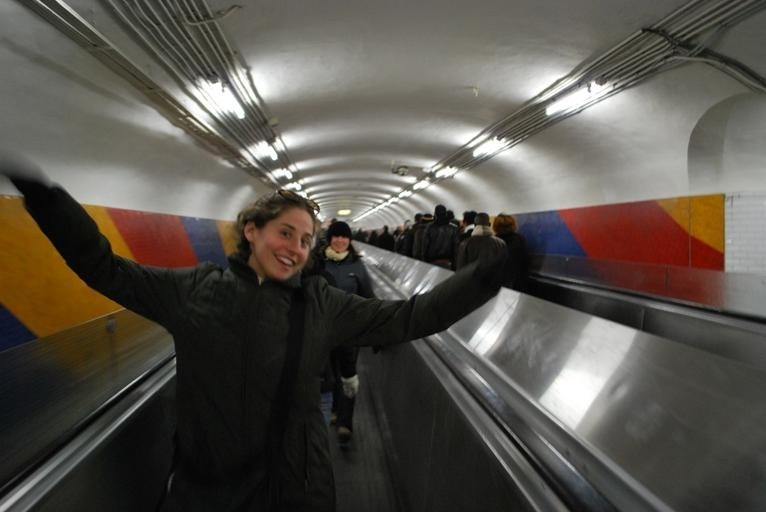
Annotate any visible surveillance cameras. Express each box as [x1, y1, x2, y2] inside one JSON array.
[[398, 167, 408, 176]]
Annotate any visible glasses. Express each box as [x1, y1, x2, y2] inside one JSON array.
[[265, 191, 321, 218]]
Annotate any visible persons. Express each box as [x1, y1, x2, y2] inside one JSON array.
[[310, 217, 380, 439], [0, 157, 512, 512], [350, 205, 530, 290]]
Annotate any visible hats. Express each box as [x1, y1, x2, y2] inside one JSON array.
[[327, 221, 351, 247]]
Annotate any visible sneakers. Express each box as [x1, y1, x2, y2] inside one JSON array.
[[330, 413, 350, 440]]
